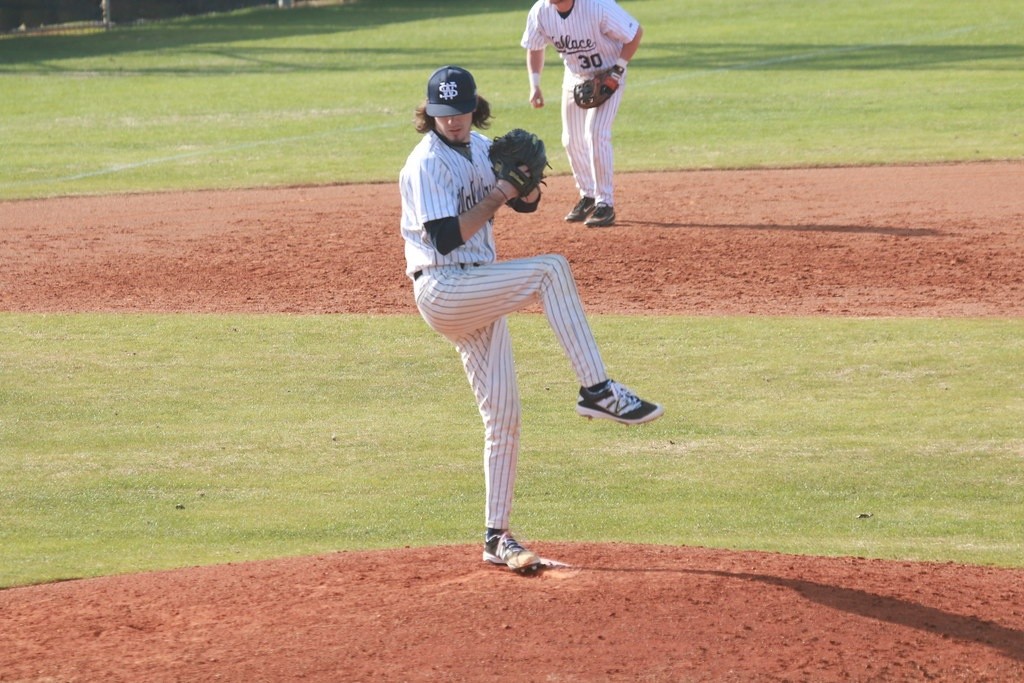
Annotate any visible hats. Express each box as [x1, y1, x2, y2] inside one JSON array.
[[425, 66, 478, 117]]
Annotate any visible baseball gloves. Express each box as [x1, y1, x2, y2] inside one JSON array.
[[488, 129, 546, 198], [573, 67, 626, 110]]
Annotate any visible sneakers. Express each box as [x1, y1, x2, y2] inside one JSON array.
[[483, 530, 540, 570], [575, 379, 664, 424], [584, 202, 615, 226], [564, 197, 595, 221]]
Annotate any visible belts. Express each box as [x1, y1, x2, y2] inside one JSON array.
[[414, 263, 479, 281]]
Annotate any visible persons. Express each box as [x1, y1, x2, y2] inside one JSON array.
[[399, 65, 665, 572], [520, 0, 642, 226]]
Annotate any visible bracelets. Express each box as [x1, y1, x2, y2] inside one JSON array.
[[495, 186, 508, 201]]
